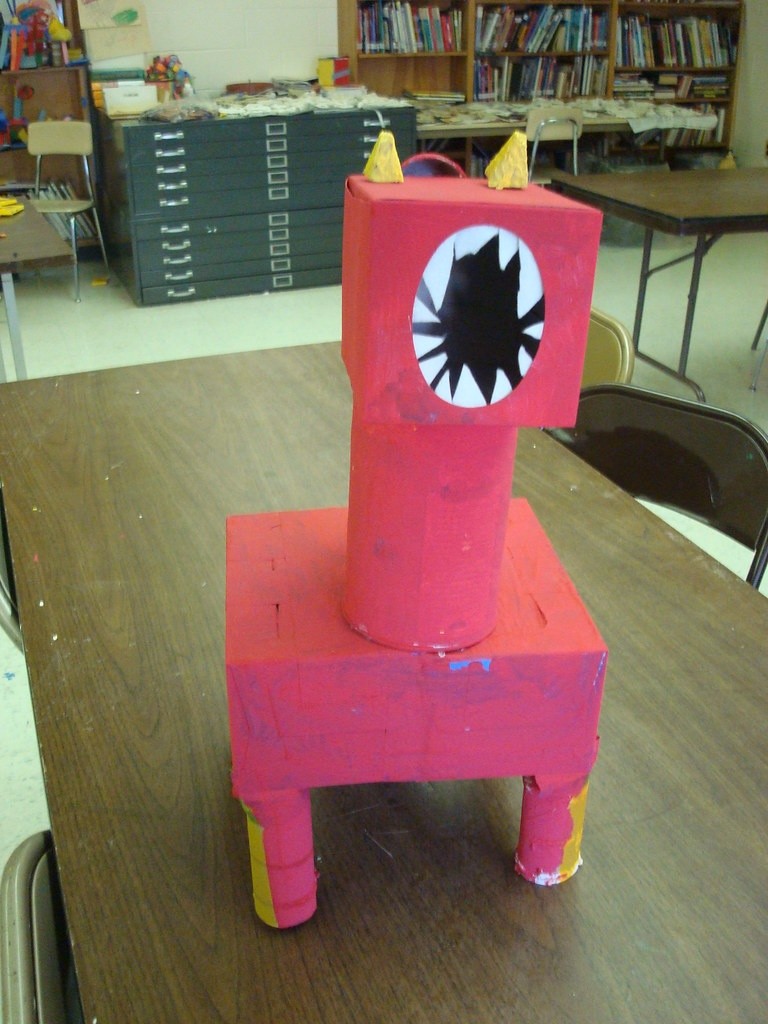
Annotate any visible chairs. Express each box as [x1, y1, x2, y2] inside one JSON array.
[[543, 382, 768, 589], [0, 829, 85, 1024], [582, 306, 636, 389], [526, 107, 584, 184], [0, 488, 23, 654], [28, 121, 112, 302]]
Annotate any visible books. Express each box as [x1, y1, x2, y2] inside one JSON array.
[[403, 88, 466, 104], [617, 12, 737, 69], [614, 72, 729, 100], [0, 180, 95, 241], [634, 104, 726, 146], [475, 4, 608, 53], [475, 56, 609, 101], [357, 0, 463, 54]]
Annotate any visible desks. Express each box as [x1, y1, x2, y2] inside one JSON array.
[[555, 167, 768, 405], [418, 104, 719, 162], [0, 339, 768, 1024], [0, 195, 77, 384]]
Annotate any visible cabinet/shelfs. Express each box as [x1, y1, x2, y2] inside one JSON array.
[[0, 65, 102, 272], [337, 0, 746, 181], [98, 89, 417, 308]]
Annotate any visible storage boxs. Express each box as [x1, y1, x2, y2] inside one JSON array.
[[103, 85, 159, 117]]
